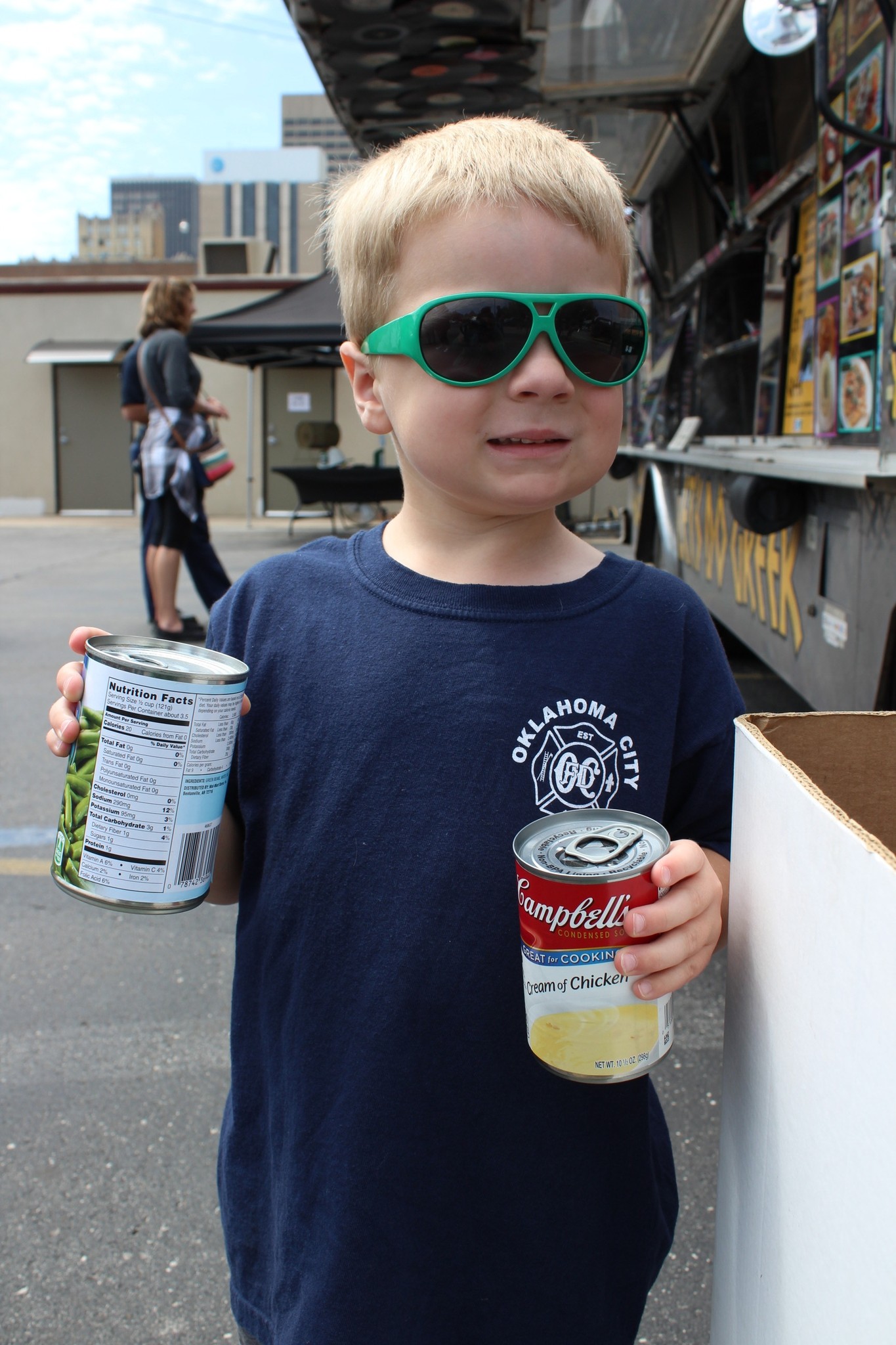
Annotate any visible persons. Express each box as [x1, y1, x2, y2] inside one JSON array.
[[115, 272, 243, 642], [44, 116, 745, 1345]]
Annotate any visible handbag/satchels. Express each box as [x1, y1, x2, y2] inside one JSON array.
[[190, 435, 238, 485]]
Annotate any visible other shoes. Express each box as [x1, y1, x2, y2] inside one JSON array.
[[182, 616, 196, 627], [156, 618, 204, 643]]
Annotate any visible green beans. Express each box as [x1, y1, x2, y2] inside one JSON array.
[[54, 706, 103, 892]]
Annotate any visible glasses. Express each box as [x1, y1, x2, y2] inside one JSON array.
[[359, 291, 650, 387]]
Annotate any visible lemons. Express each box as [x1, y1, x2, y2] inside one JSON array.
[[530, 1005, 661, 1076]]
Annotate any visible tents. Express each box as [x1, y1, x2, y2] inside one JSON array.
[[184, 263, 382, 530]]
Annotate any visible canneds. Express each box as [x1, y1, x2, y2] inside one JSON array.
[[511, 809, 677, 1082], [51, 634, 249, 917]]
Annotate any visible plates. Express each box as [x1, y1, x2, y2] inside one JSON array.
[[839, 358, 876, 431], [816, 351, 836, 432], [818, 194, 872, 343]]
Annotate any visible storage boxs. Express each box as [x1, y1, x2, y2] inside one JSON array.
[[709, 711, 896, 1343]]
